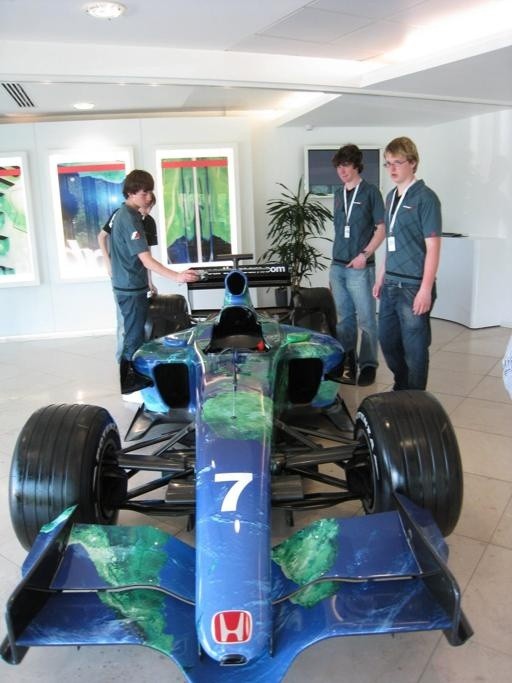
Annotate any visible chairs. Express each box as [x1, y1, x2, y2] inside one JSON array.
[[209, 306, 270, 353]]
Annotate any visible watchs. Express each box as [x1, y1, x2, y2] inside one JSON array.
[[360, 250, 370, 258]]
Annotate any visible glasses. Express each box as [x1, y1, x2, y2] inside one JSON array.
[[382, 159, 408, 169]]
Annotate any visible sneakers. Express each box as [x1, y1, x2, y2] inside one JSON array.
[[358, 366, 375, 386]]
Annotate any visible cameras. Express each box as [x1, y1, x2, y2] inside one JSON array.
[[196, 272, 209, 282]]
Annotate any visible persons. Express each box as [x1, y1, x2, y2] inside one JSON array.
[[96, 192, 158, 363], [371, 138, 443, 391], [110, 168, 201, 361], [328, 143, 387, 386]]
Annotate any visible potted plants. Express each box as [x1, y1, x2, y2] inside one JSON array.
[[257, 174, 334, 307]]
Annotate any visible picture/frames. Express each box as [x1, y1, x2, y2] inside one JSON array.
[[0, 142, 239, 289]]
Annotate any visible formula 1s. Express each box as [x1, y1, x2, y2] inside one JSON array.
[[0, 255, 475, 683]]
